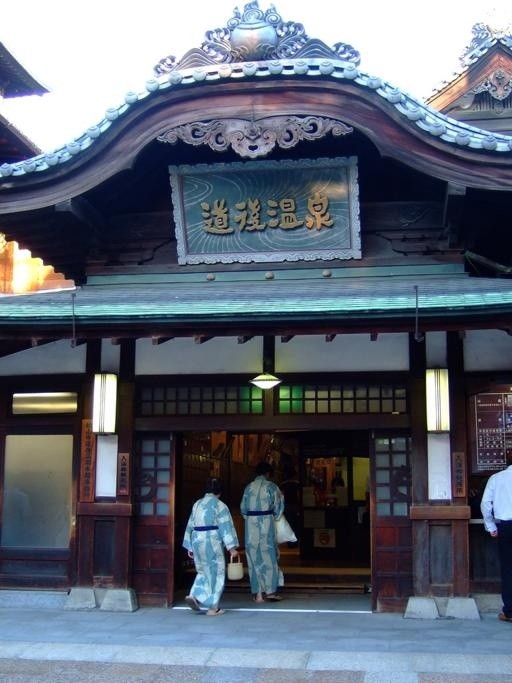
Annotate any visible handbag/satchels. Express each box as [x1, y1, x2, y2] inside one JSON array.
[[226, 563, 244, 581]]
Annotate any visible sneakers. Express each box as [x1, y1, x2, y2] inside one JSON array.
[[498, 612, 512, 621]]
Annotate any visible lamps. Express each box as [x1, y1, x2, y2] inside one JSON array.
[[248, 373, 283, 390], [423, 366, 452, 434], [91, 372, 119, 435]]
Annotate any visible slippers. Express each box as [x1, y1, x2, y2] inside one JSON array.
[[206, 607, 226, 616], [253, 595, 282, 602], [185, 595, 200, 611]]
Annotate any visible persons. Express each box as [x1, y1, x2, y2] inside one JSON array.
[[279, 470, 301, 548], [481, 454, 511, 621], [182, 479, 240, 617], [240, 462, 284, 603]]
